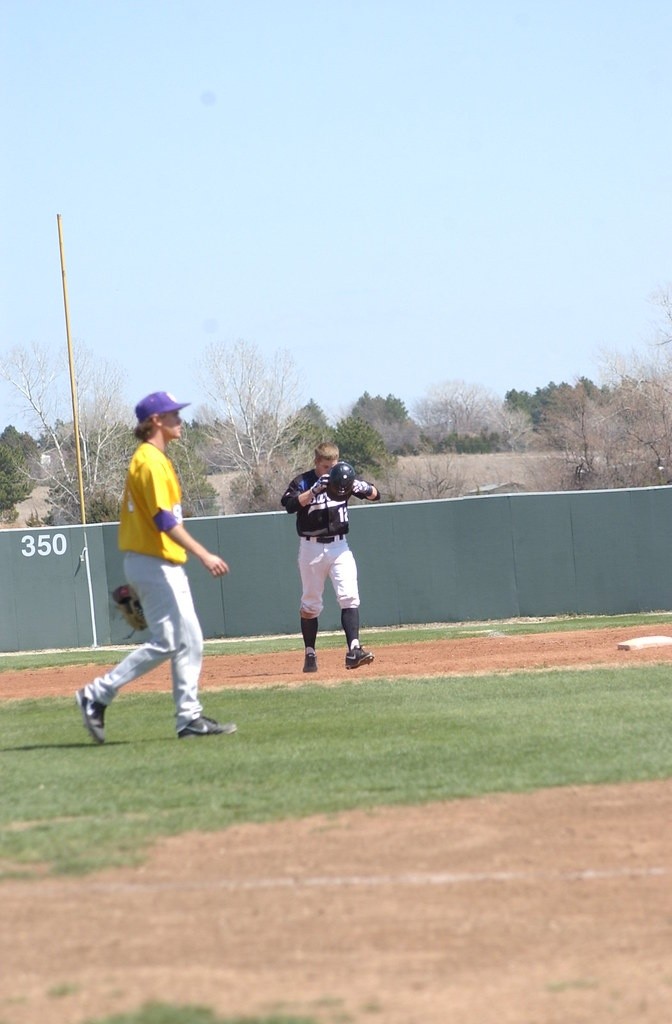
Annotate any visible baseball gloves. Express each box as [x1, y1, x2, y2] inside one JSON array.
[[109, 583, 148, 641]]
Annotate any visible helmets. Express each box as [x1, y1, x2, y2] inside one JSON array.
[[327, 462, 355, 501]]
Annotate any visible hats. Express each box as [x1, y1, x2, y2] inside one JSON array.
[[136, 392, 190, 421]]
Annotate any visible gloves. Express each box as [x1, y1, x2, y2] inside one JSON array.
[[310, 474, 331, 495], [352, 479, 370, 495]]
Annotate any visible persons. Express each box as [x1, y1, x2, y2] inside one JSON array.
[[280, 443, 381, 672], [73, 392, 238, 745]]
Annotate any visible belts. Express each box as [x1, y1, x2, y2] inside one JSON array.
[[306, 535, 343, 543]]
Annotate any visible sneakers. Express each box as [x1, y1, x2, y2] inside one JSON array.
[[178, 716, 236, 736], [345, 647, 375, 669], [76, 688, 106, 743], [303, 653, 318, 672]]
